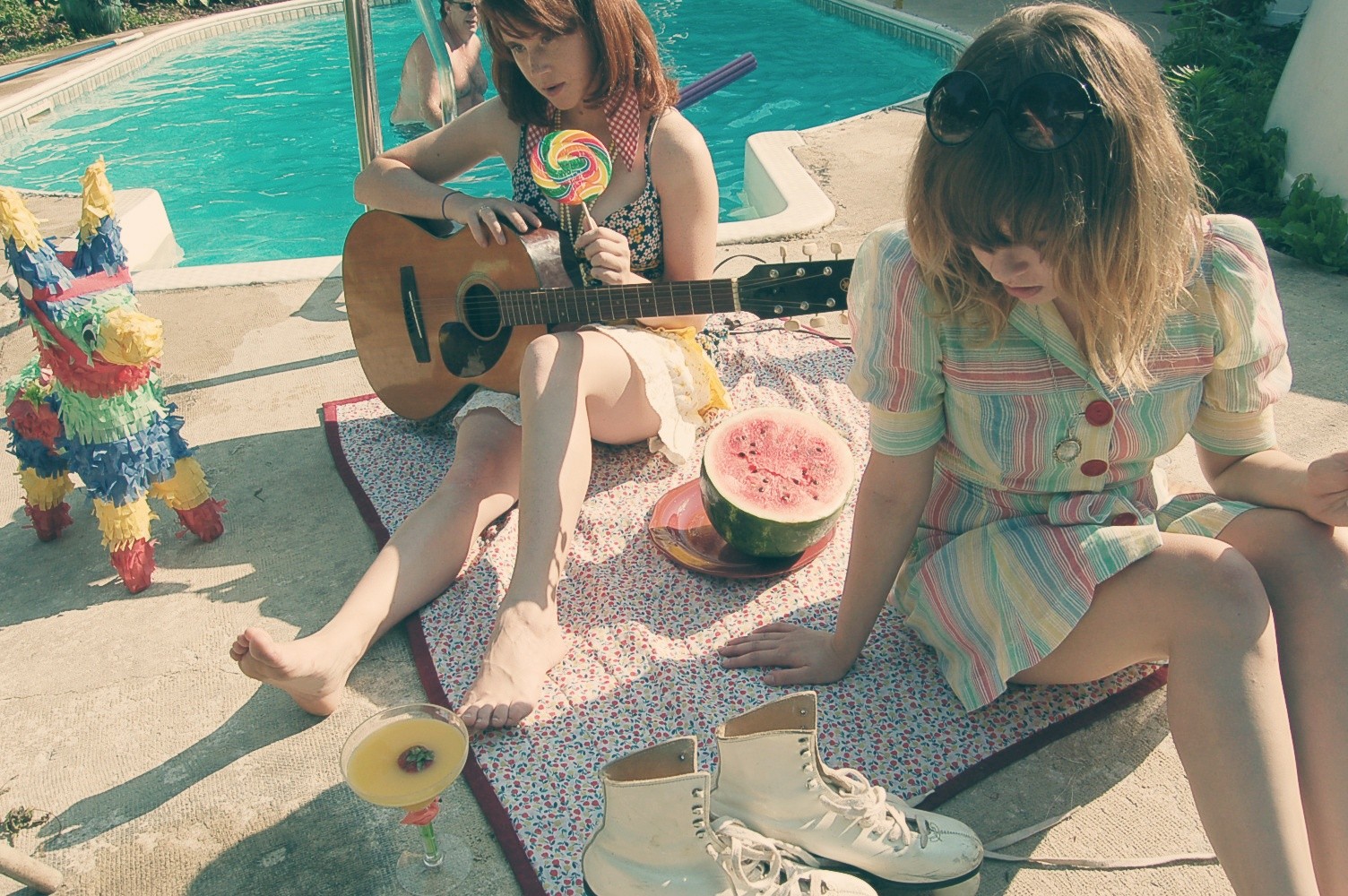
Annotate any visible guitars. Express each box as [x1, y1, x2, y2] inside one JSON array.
[[342, 210, 855, 421]]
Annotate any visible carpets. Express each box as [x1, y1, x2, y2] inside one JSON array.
[[312, 312, 1174, 896]]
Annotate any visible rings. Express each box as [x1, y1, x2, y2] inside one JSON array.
[[479, 205, 490, 215]]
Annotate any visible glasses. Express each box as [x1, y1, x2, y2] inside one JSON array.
[[453, 1, 473, 10], [922, 70, 1105, 150]]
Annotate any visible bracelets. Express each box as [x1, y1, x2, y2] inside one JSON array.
[[442, 190, 462, 221]]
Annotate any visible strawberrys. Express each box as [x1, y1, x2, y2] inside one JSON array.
[[397, 746, 435, 772]]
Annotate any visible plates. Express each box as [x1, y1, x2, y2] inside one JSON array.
[[647, 476, 837, 578]]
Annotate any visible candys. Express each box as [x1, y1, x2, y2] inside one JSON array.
[[531, 129, 612, 231]]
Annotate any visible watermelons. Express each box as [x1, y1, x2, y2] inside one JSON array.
[[700, 407, 855, 558]]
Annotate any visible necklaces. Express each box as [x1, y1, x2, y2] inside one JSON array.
[[552, 108, 618, 286], [1036, 304, 1091, 465]]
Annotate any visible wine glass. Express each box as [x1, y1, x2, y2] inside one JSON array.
[[338, 702, 474, 896]]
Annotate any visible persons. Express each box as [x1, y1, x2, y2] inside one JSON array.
[[390, 1, 489, 125], [718, 2, 1348, 896], [229, 0, 719, 730]]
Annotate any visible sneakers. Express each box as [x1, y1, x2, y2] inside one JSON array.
[[707, 691, 983, 895], [581, 736, 879, 896]]
